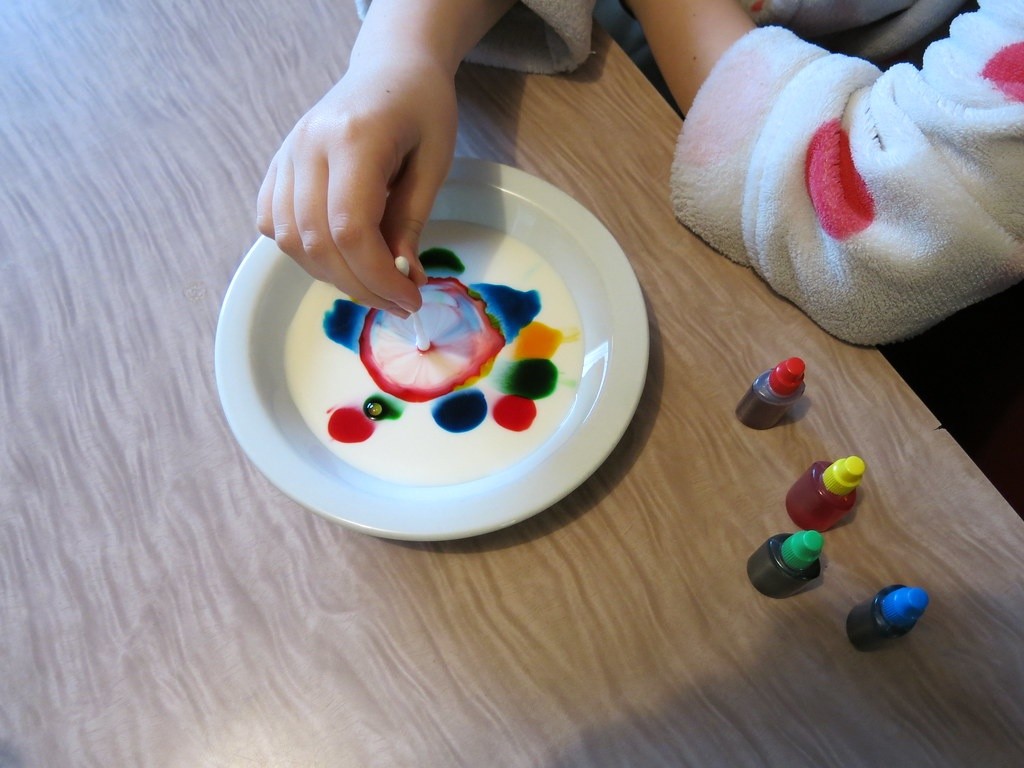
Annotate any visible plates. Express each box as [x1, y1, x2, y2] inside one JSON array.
[[214, 159, 651, 539]]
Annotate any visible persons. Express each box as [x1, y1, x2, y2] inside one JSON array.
[[255, 0, 1024, 348]]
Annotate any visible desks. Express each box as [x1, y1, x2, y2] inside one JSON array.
[[0, 0, 1024, 768]]
